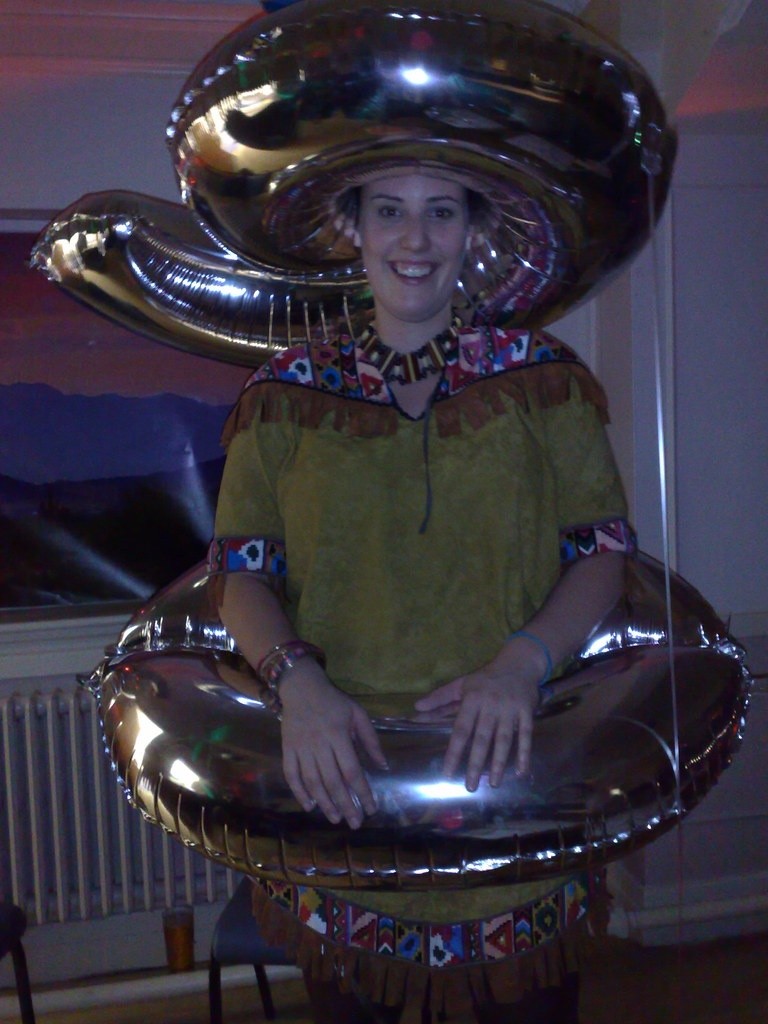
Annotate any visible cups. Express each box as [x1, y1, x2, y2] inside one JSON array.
[[161, 905, 195, 973]]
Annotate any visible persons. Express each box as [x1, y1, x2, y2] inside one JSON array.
[[202, 175, 636, 1024]]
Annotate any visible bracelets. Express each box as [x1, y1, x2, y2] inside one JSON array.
[[505, 631, 553, 686], [258, 640, 327, 701]]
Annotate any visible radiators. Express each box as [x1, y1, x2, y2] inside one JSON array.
[[1, 650, 242, 983]]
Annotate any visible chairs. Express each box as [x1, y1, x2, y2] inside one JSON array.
[[0, 902, 36, 1023], [209, 876, 296, 1024]]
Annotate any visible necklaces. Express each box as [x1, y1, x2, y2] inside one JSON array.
[[360, 316, 462, 383]]
